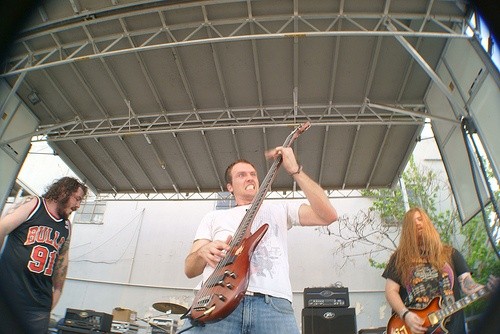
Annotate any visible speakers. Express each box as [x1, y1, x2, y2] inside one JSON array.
[[302, 307, 357, 334]]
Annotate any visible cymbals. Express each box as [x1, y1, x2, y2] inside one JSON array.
[[152, 303, 188, 314]]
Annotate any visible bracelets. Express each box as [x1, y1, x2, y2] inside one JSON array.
[[290, 165, 303, 177], [402, 310, 410, 321]]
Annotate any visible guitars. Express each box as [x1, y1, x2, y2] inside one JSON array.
[[386, 285, 493, 334], [190, 121, 312, 324]]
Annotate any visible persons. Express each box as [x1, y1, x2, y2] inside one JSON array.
[[177, 146, 339, 334], [382, 207, 498, 334], [0, 176, 89, 334]]
